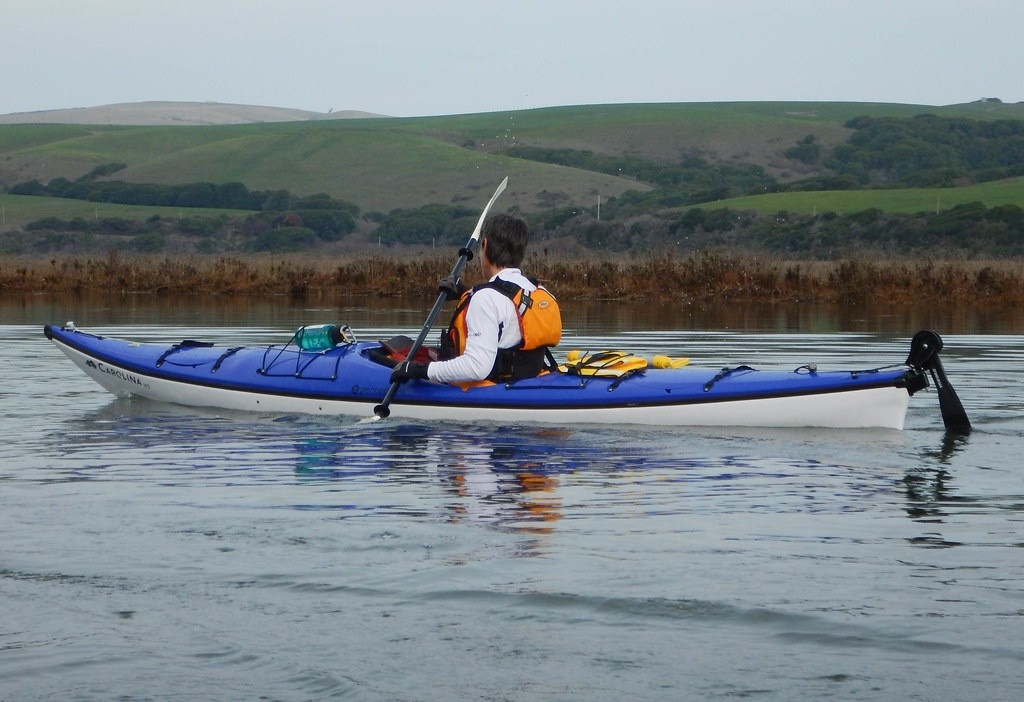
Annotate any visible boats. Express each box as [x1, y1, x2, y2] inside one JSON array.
[[45, 322, 915, 433]]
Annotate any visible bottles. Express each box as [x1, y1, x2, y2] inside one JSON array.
[[294, 324, 344, 350]]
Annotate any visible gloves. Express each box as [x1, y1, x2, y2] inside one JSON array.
[[435, 274, 470, 301], [389, 360, 430, 387]]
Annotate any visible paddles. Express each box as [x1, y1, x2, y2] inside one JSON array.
[[373, 175, 509, 418]]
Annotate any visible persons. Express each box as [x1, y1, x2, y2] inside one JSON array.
[[388, 213, 548, 385]]
